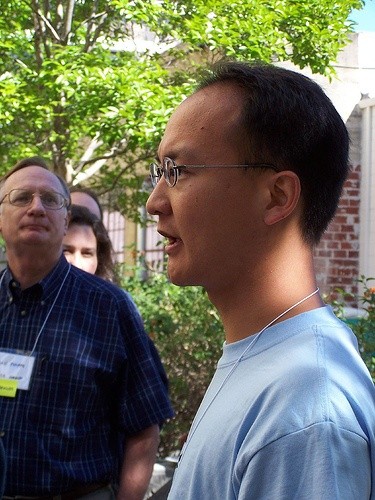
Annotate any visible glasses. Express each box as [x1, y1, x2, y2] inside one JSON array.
[[0, 188, 70, 210], [149, 157, 281, 189]]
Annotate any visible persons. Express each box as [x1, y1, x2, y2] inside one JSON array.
[[143, 65, 375, 500], [59, 182, 119, 286], [0, 157, 172, 500]]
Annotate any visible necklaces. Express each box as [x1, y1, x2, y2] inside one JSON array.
[[181, 287, 319, 459]]
